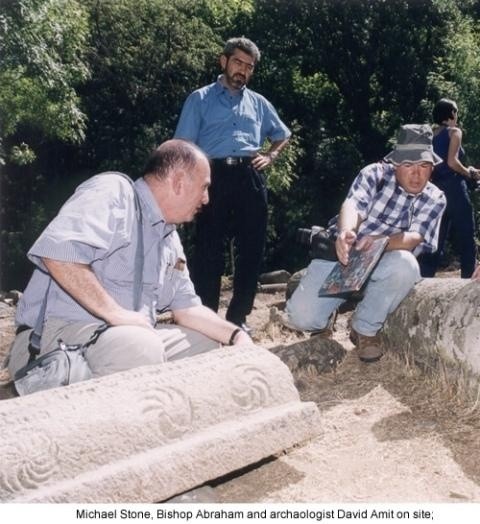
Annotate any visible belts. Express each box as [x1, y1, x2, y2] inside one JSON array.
[[211, 157, 252, 168]]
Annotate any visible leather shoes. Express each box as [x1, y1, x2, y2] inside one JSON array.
[[310, 308, 339, 337], [350, 329, 383, 360]]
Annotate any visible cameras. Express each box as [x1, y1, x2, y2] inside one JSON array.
[[294, 225, 338, 260]]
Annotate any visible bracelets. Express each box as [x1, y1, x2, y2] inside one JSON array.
[[470, 169, 474, 178], [266, 150, 278, 158], [229, 328, 244, 346]]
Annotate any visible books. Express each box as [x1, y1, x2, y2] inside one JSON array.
[[317, 236, 392, 298]]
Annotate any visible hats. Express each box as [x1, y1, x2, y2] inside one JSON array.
[[383, 124, 443, 167]]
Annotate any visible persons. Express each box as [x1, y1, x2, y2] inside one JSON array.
[[421, 98, 480, 279], [285, 124, 447, 361], [1, 139, 256, 396], [174, 35, 291, 324]]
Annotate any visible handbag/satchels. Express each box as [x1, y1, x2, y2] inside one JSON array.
[[13, 338, 92, 397], [309, 213, 340, 262]]
